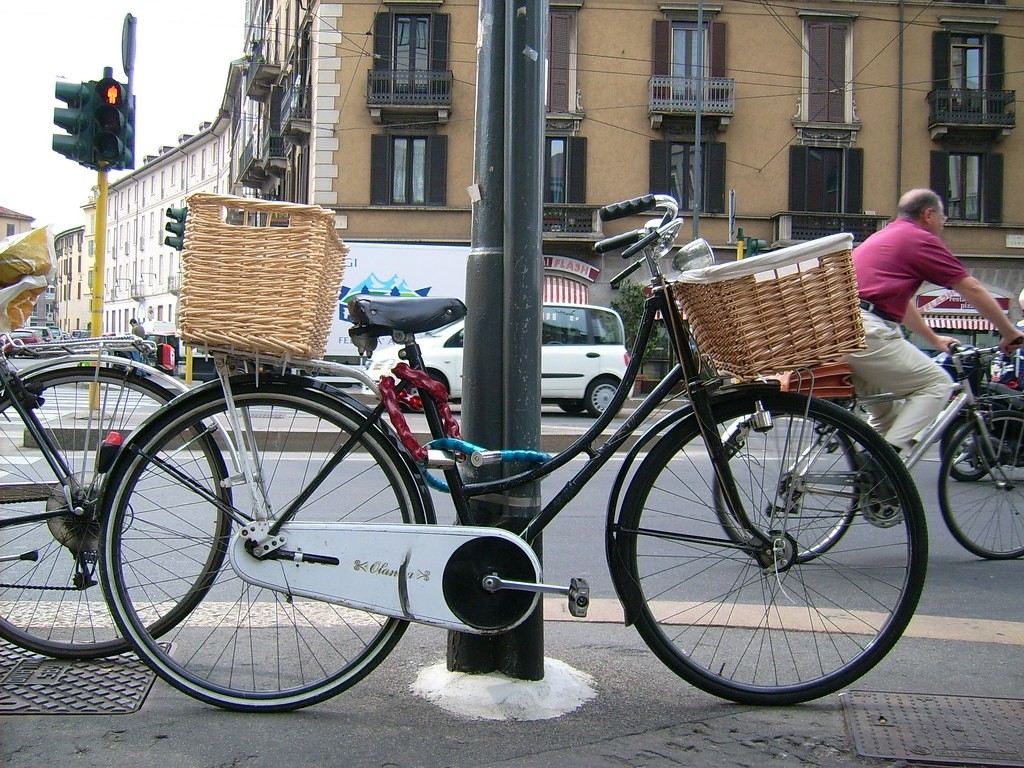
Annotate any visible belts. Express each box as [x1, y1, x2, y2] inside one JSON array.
[[859, 300, 900, 323]]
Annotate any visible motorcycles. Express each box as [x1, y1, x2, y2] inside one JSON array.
[[939, 380, 1024, 481]]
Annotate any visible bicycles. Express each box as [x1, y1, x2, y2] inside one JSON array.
[[713, 337, 1024, 564], [93, 189, 929, 715], [0, 226, 233, 659]]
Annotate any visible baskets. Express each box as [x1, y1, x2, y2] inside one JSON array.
[[176, 191, 350, 360], [672, 233, 867, 383]]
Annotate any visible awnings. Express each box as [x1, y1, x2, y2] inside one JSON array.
[[544, 274, 588, 304], [922, 317, 1000, 331]]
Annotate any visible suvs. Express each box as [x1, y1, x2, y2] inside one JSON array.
[[47, 327, 65, 343], [24, 326, 53, 350], [5, 329, 42, 359]]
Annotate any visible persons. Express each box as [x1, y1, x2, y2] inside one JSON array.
[[1011, 288, 1024, 389], [834, 189, 1024, 507], [129, 318, 147, 379]]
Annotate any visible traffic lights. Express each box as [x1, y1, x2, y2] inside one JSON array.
[[164, 206, 187, 251], [91, 78, 131, 165], [52, 80, 93, 163]]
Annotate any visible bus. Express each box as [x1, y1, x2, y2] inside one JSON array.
[[72, 330, 89, 339]]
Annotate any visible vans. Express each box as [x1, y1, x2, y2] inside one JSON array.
[[366, 302, 636, 420], [145, 333, 179, 366]]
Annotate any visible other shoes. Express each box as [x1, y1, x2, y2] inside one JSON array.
[[855, 450, 898, 505]]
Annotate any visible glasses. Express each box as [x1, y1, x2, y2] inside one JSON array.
[[933, 210, 948, 224]]
